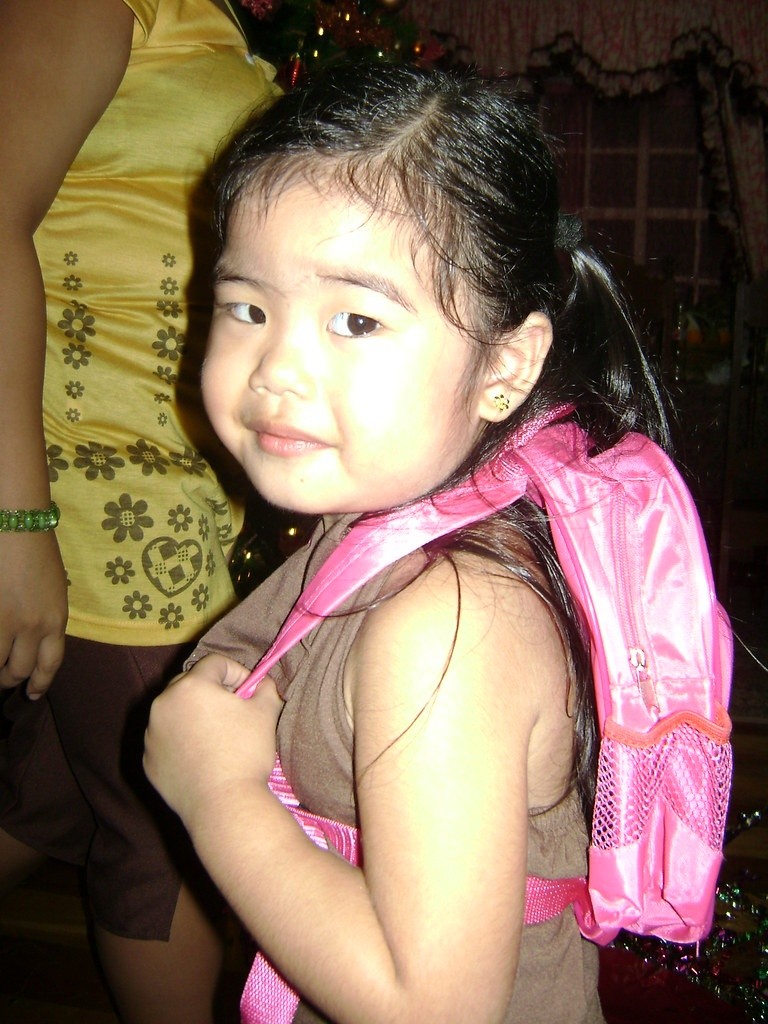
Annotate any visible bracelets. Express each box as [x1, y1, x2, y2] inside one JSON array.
[[1, 501, 60, 534]]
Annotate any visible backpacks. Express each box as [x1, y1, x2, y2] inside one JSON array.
[[230, 402, 735, 1024]]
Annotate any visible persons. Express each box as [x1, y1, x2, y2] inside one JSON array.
[[0, 0, 282, 1024], [144, 65, 604, 1024]]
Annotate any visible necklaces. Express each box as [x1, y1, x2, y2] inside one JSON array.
[[225, 1, 252, 55]]
